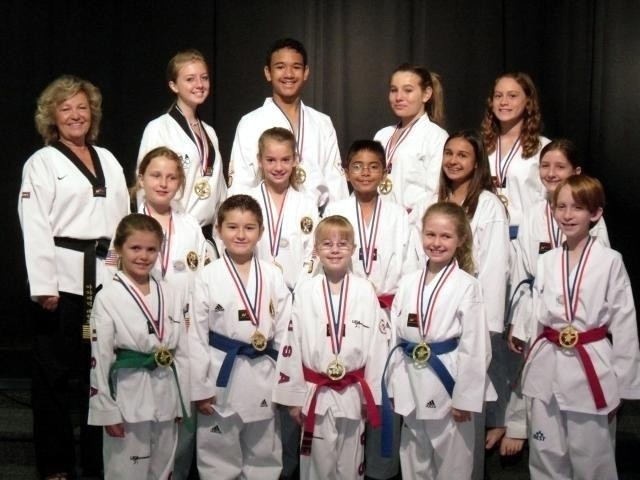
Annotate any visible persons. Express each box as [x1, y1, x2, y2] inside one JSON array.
[[136, 51, 226, 480], [189, 39, 388, 480], [88, 213, 192, 480], [480, 71, 640, 480], [17, 74, 133, 480], [330, 64, 507, 480]]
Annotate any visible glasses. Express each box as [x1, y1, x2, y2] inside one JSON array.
[[349, 165, 384, 173], [316, 238, 354, 251]]
